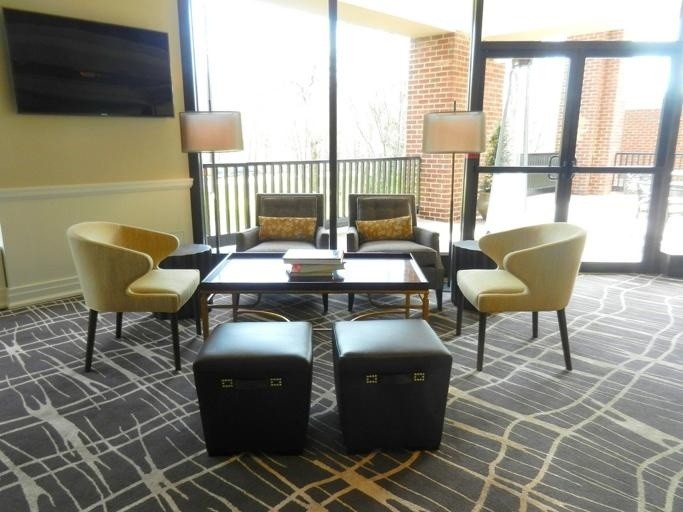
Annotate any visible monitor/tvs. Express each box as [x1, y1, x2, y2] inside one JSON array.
[[2, 7, 174, 117]]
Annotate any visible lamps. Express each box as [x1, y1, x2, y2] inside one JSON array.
[[180, 100, 244, 264], [422, 101, 486, 292]]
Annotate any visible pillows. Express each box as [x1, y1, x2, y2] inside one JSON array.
[[258, 215, 318, 241], [355, 216, 414, 240]]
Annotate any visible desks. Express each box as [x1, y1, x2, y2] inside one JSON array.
[[200, 252, 430, 342]]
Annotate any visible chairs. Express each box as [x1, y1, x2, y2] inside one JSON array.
[[456, 223, 584, 371], [233, 194, 330, 314], [344, 193, 445, 310], [68, 221, 199, 371]]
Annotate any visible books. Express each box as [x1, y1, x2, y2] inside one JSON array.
[[285, 268, 343, 282], [282, 249, 344, 264], [288, 262, 345, 273]]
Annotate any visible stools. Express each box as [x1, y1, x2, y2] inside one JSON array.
[[331, 319, 453, 455], [153, 244, 211, 319], [451, 239, 496, 316], [193, 322, 312, 457]]
